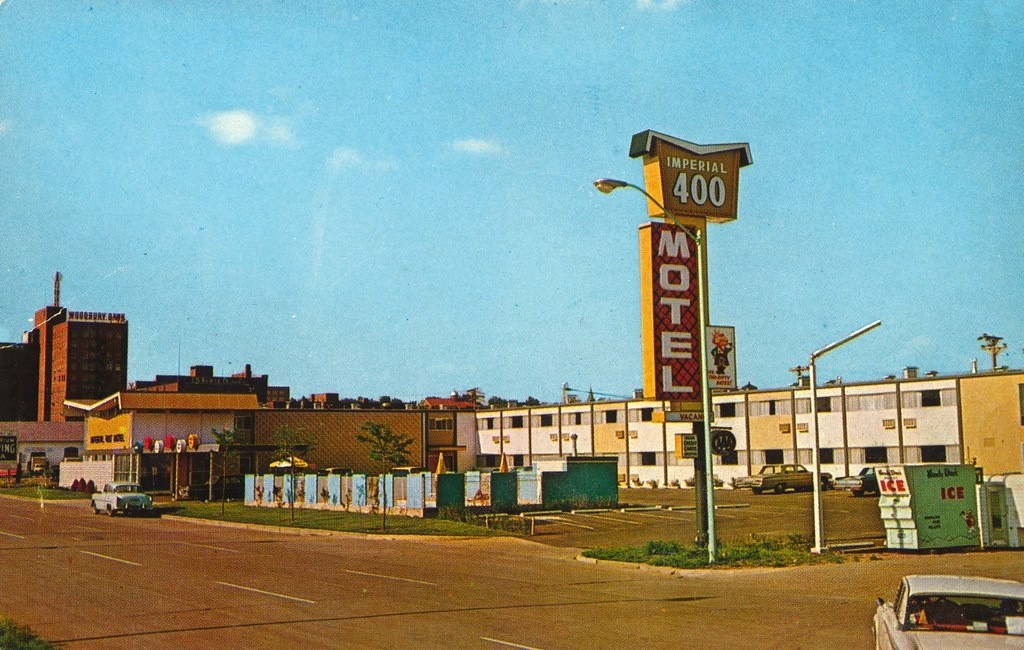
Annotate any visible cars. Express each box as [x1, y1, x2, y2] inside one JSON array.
[[872, 574, 1024, 650], [833, 466, 881, 497], [735, 464, 833, 494], [90, 482, 154, 518]]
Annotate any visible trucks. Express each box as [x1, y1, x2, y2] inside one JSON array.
[[30, 457, 46, 474]]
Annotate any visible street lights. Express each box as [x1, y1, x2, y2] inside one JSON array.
[[593, 178, 717, 565]]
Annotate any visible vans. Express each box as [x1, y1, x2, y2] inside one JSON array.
[[388, 467, 428, 478], [317, 468, 353, 477]]
